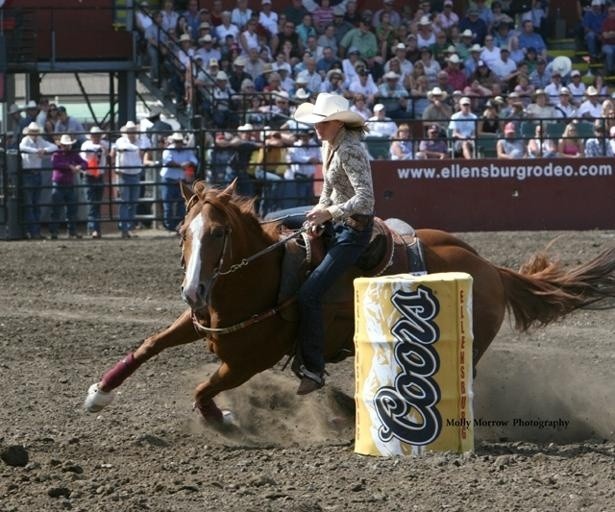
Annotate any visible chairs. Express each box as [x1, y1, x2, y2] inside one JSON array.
[[135, 2, 615, 168]]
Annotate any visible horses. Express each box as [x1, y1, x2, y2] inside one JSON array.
[[84, 177, 615, 437]]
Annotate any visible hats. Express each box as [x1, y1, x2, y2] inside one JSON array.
[[177, 1, 605, 136], [6, 96, 185, 145]]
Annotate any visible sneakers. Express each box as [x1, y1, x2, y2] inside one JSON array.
[[296, 371, 323, 395]]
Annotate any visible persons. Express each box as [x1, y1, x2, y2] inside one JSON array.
[[0, 1, 614, 239], [263, 92, 374, 395]]
